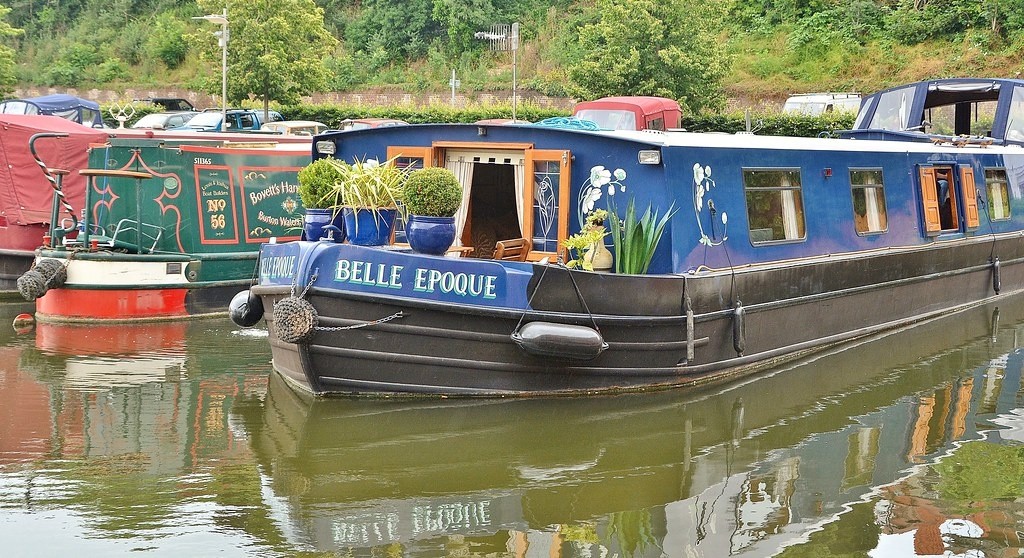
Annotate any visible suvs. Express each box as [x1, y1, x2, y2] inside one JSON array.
[[167, 108, 286, 134]]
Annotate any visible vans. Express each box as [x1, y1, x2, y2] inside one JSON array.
[[780, 93, 863, 121]]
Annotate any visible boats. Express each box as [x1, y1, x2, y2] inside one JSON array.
[[17, 95, 684, 319], [0, 111, 153, 302], [255, 288, 1024, 558], [251, 78, 1024, 400]]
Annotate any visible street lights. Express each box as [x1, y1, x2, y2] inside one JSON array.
[[204, 9, 230, 132]]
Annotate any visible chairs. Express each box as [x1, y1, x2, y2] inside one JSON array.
[[494, 238, 530, 262]]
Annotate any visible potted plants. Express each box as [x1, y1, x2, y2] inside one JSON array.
[[297, 151, 417, 246], [401, 167, 462, 256], [584, 208, 613, 269]]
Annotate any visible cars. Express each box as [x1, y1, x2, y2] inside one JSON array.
[[135, 111, 202, 133]]
